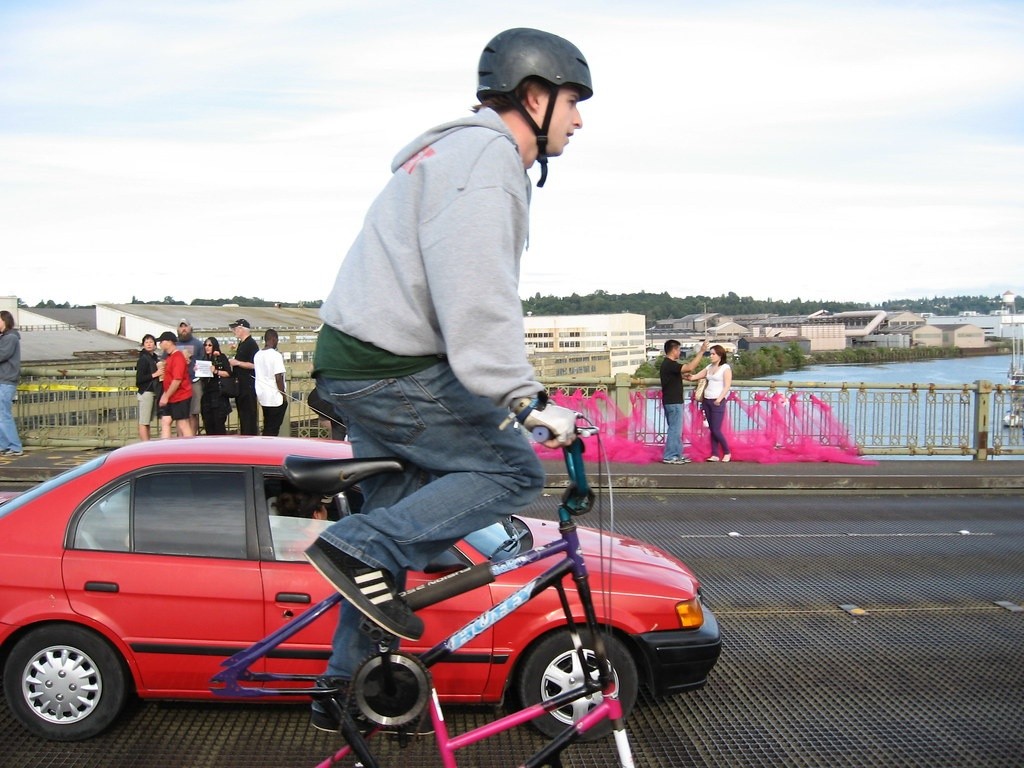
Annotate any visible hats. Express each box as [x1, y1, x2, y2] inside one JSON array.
[[155, 331, 178, 342], [229, 318, 251, 329], [178, 318, 190, 326]]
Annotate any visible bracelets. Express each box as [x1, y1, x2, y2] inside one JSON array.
[[214, 370, 218, 375], [235, 361, 240, 367]]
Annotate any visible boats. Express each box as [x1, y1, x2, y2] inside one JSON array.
[[1002, 331, 1024, 429]]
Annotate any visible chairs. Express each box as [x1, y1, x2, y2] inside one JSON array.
[[267, 493, 297, 519]]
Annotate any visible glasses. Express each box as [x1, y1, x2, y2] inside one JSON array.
[[204, 344, 212, 347]]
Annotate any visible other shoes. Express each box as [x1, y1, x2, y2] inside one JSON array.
[[1, 448, 22, 457], [706, 456, 720, 461], [663, 456, 691, 464], [722, 453, 731, 462]]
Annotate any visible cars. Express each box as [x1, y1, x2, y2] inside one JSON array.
[[0, 435, 720, 741]]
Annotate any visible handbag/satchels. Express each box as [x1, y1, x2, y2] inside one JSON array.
[[217, 377, 239, 397], [694, 378, 707, 402]]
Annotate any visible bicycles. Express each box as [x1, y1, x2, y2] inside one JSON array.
[[207, 413, 637, 768]]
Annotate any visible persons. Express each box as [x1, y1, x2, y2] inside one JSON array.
[[135, 317, 206, 440], [0, 311, 24, 456], [193, 337, 232, 435], [279, 489, 329, 560], [332, 415, 348, 442], [683, 345, 732, 462], [660, 340, 709, 465], [304, 29, 593, 735], [254, 329, 288, 437], [228, 318, 260, 435]]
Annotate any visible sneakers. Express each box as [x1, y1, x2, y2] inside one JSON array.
[[312, 708, 433, 735], [304, 537, 425, 641]]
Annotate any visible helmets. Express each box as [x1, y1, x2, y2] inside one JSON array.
[[476, 27, 593, 102]]
